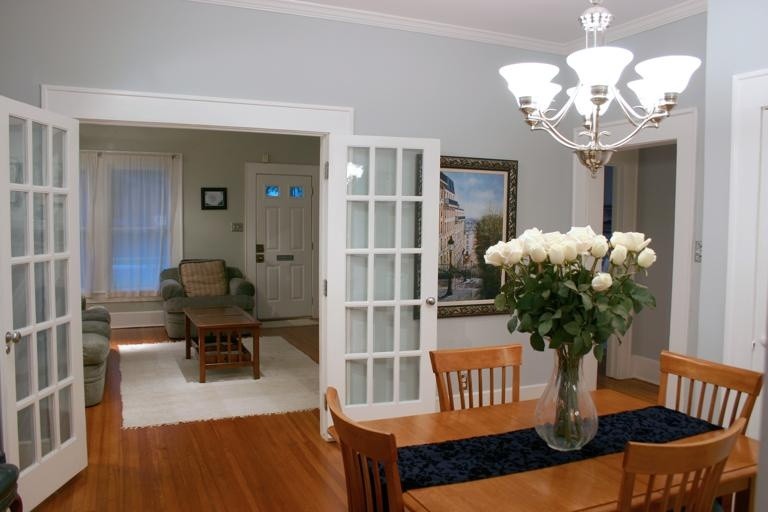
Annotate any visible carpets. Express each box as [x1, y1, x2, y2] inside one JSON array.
[[118, 335, 319, 428]]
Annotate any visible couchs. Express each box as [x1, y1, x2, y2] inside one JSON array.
[[160, 258, 255, 337], [80, 296, 110, 406]]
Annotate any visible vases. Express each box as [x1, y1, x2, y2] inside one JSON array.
[[533, 342, 598, 450]]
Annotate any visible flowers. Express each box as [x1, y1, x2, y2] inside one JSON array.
[[484, 225, 658, 444]]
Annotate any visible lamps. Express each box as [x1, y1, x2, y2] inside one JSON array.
[[498, 0, 701, 179]]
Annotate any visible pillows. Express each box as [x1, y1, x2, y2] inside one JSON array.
[[179, 262, 227, 297], [180, 259, 226, 262]]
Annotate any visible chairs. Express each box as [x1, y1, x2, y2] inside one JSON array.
[[656, 350, 763, 434], [326, 386, 404, 512], [429, 344, 521, 412], [617, 418, 747, 512]]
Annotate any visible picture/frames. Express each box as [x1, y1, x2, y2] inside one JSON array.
[[201, 187, 227, 210], [414, 153, 518, 320]]
